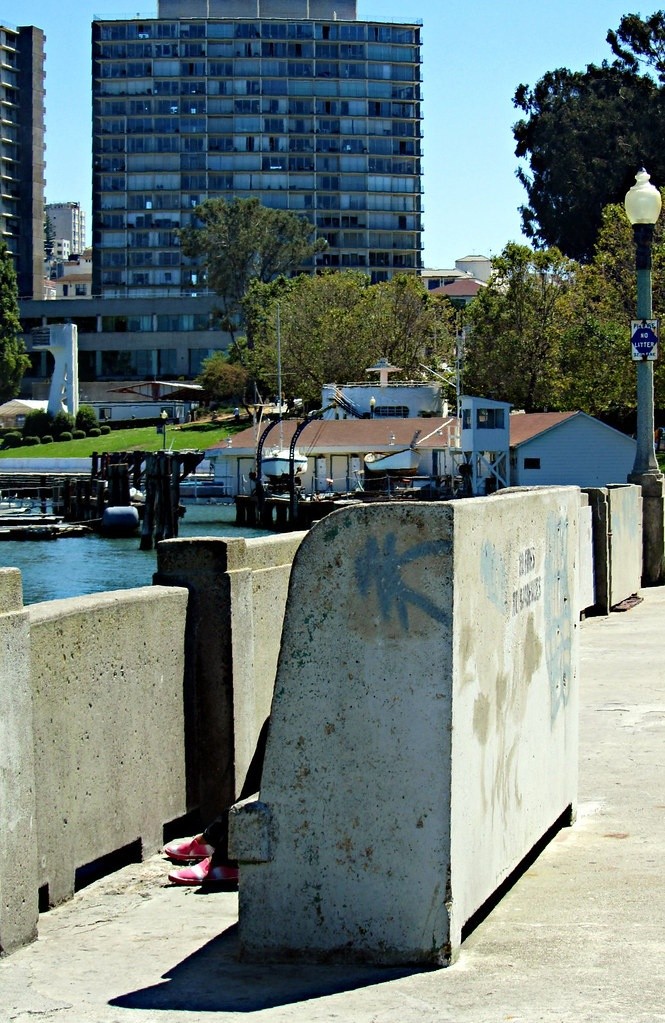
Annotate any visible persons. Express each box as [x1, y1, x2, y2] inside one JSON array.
[[282, 390, 285, 406], [233, 406, 239, 422], [162, 713, 271, 885], [273, 393, 278, 406]]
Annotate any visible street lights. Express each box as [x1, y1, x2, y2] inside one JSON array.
[[624, 167, 663, 473], [370, 396, 375, 420], [162, 410, 168, 449]]
